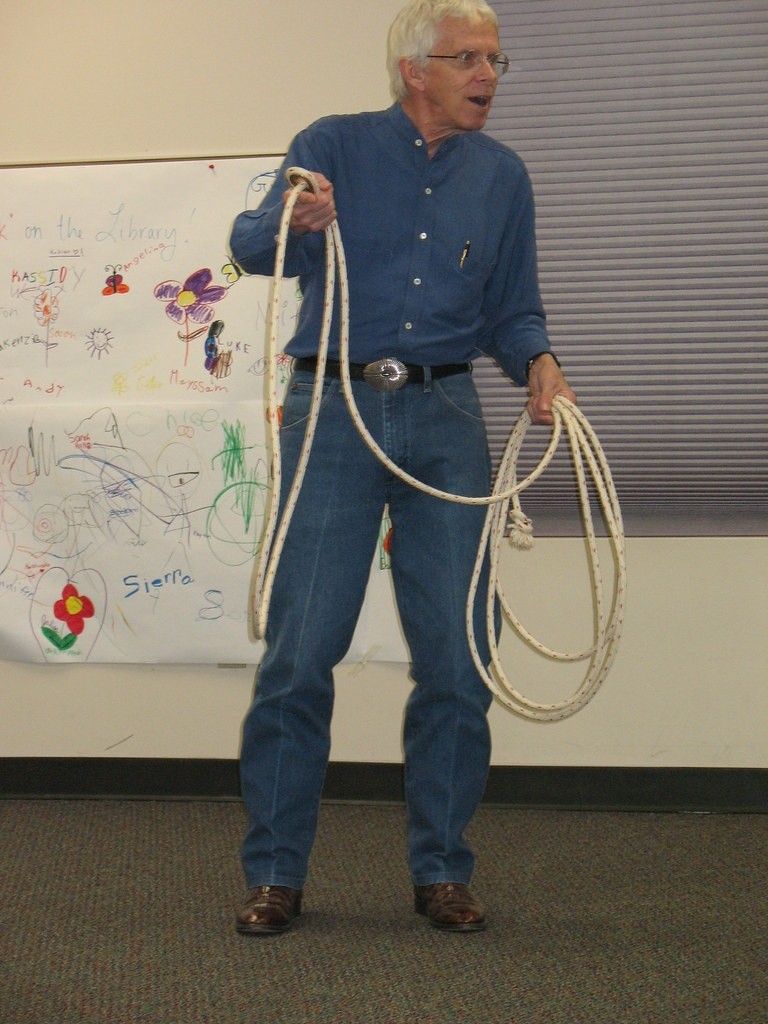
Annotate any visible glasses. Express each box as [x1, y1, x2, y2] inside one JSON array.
[[424, 49, 512, 78]]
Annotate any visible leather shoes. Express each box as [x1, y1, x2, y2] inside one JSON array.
[[236, 884, 302, 934], [413, 881, 488, 932]]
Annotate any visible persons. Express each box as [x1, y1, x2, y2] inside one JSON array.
[[229, 0, 575, 934]]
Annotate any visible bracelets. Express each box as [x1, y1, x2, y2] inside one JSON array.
[[526, 359, 534, 378]]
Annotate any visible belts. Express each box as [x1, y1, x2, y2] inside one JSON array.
[[292, 356, 473, 392]]
